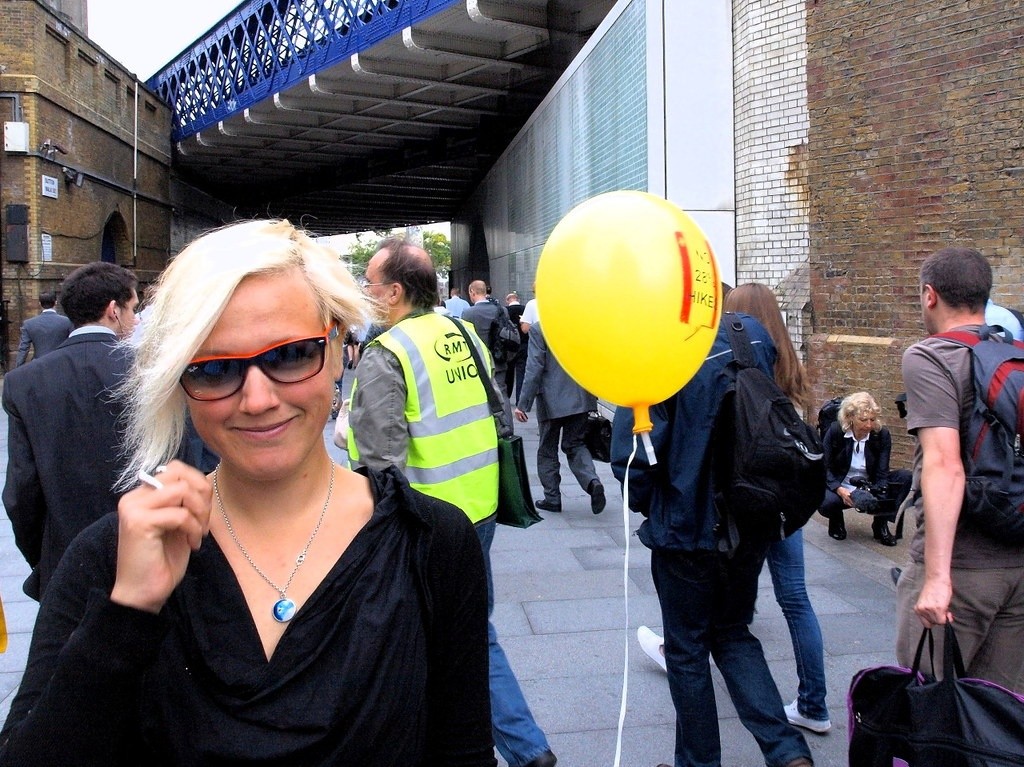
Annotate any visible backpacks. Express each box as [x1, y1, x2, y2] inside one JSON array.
[[488, 302, 520, 361], [815, 396, 848, 441], [717, 310, 826, 542], [925, 324, 1024, 545]]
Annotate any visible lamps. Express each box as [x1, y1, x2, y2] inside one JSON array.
[[62, 167, 84, 188]]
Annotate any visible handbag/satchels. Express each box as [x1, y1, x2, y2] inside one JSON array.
[[584, 411, 612, 463], [447, 313, 542, 530], [847, 613, 1024, 767]]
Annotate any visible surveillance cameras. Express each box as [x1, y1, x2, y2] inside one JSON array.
[[52, 142, 69, 155], [44, 138, 51, 148], [76, 172, 84, 187]]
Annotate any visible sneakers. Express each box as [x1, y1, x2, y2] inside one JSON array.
[[636, 625, 668, 673], [783, 699, 831, 732]]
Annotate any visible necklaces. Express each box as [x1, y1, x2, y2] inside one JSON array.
[[213, 454, 334, 623]]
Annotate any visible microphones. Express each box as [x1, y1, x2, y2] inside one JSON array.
[[850, 488, 877, 510]]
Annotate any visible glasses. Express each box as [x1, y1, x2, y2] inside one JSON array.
[[361, 277, 388, 292], [179, 314, 341, 400]]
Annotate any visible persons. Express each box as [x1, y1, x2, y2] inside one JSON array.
[[609, 283, 830, 767], [817, 392, 913, 547], [16, 292, 76, 368], [178, 408, 220, 476], [331, 280, 539, 420], [0, 218, 498, 767], [893, 248, 1024, 767], [134, 298, 155, 332], [349, 236, 556, 767], [515, 322, 606, 514], [2, 263, 203, 605]]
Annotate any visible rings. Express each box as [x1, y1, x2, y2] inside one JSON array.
[[138, 470, 163, 489], [152, 466, 167, 476], [519, 417, 522, 418]]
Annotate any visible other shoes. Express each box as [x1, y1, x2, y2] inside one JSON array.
[[591, 479, 606, 514], [535, 500, 562, 512], [872, 523, 898, 546], [829, 513, 846, 540]]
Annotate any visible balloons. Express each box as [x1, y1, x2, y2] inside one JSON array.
[[536, 191, 723, 433]]
